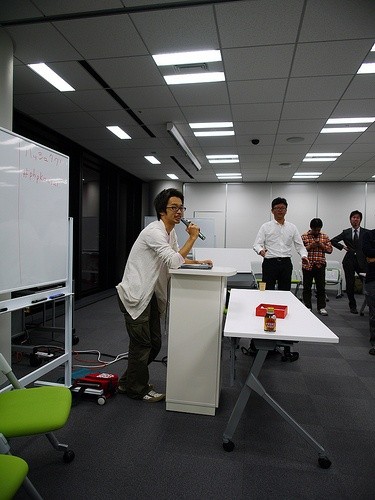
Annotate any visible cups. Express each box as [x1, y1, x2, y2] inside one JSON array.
[[259, 282, 266, 291]]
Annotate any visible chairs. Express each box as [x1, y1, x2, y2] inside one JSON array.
[[251, 261, 342, 302], [0, 353, 71, 500]]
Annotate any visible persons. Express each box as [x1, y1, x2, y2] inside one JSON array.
[[301, 218, 333, 316], [116, 188, 212, 402], [362, 228, 375, 354], [253, 197, 309, 291], [331, 210, 368, 314]]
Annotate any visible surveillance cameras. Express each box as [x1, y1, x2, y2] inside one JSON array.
[[252, 139, 259, 144]]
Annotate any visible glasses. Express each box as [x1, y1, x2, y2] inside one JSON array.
[[273, 207, 287, 211], [163, 205, 186, 212]]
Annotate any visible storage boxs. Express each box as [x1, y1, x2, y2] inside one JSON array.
[[256, 304, 288, 319]]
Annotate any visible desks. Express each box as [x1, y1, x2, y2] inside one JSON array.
[[216, 288, 339, 469]]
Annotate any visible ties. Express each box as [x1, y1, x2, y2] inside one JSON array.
[[354, 229, 359, 249]]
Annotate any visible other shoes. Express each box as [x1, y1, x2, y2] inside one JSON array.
[[117, 384, 164, 402], [350, 307, 359, 314], [318, 308, 328, 316]]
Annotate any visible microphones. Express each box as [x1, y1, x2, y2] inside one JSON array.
[[181, 218, 206, 240]]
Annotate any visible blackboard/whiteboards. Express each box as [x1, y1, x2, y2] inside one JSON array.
[[0, 127, 70, 294]]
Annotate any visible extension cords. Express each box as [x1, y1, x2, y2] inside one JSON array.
[[35, 352, 53, 357]]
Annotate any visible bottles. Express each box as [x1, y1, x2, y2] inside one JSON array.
[[263, 306, 277, 331]]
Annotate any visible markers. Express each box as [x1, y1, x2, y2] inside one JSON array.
[[31, 298, 47, 303], [0, 308, 8, 312], [50, 293, 65, 300]]
